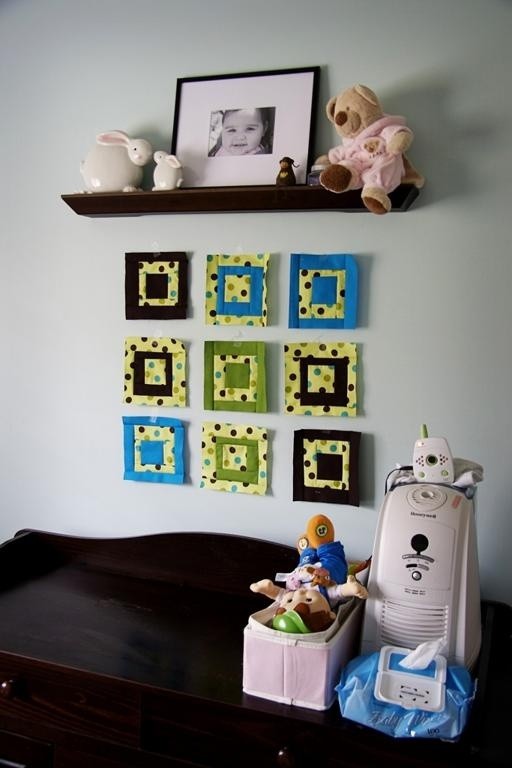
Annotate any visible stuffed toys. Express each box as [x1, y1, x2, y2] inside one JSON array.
[[276, 155, 300, 185], [312, 83, 425, 215]]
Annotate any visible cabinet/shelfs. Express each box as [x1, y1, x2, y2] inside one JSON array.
[[0, 527, 512, 768]]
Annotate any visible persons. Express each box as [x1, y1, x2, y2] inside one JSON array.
[[249, 513, 370, 637], [207, 108, 271, 155]]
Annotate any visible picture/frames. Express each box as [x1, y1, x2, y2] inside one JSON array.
[[171, 66, 321, 191]]
